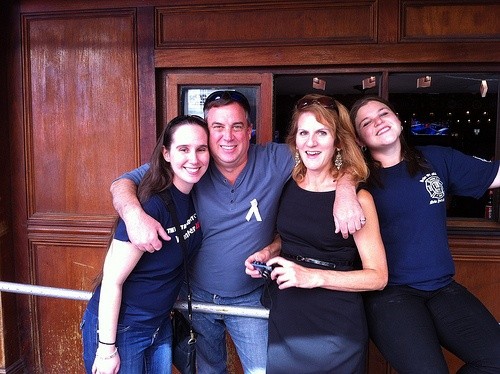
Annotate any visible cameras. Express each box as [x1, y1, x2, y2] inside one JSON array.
[[251, 260, 278, 280]]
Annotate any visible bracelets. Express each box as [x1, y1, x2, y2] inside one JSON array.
[[99, 341, 116, 345], [95, 349, 117, 360]]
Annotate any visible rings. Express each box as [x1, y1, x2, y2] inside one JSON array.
[[362, 218, 366, 221]]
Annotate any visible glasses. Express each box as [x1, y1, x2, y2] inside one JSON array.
[[204, 91, 248, 109], [297, 96, 339, 115], [168, 115, 206, 131]]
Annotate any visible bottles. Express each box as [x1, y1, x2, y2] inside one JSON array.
[[486, 190, 493, 220]]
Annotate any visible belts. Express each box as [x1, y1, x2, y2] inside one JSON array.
[[279, 252, 336, 268]]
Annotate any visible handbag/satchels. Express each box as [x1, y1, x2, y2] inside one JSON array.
[[170, 308, 198, 374]]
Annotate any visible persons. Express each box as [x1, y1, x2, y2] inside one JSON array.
[[82, 115, 210, 374], [350, 96, 500, 374], [110, 92, 366, 374], [244, 94, 388, 374]]
